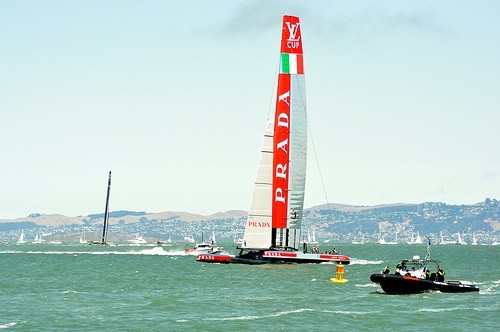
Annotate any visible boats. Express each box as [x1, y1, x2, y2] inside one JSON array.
[[185, 242, 222, 254], [352, 236, 365, 244], [370, 239, 479, 292], [377, 231, 423, 244], [126, 236, 147, 243]]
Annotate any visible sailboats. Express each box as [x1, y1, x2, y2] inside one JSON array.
[[457, 231, 467, 245], [196, 16, 350, 264], [80, 229, 87, 243], [32, 233, 61, 244], [16, 228, 26, 243], [438, 232, 446, 244], [472, 231, 478, 245]]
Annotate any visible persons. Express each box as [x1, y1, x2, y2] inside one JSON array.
[[311, 246, 338, 255], [381, 264, 446, 283]]
